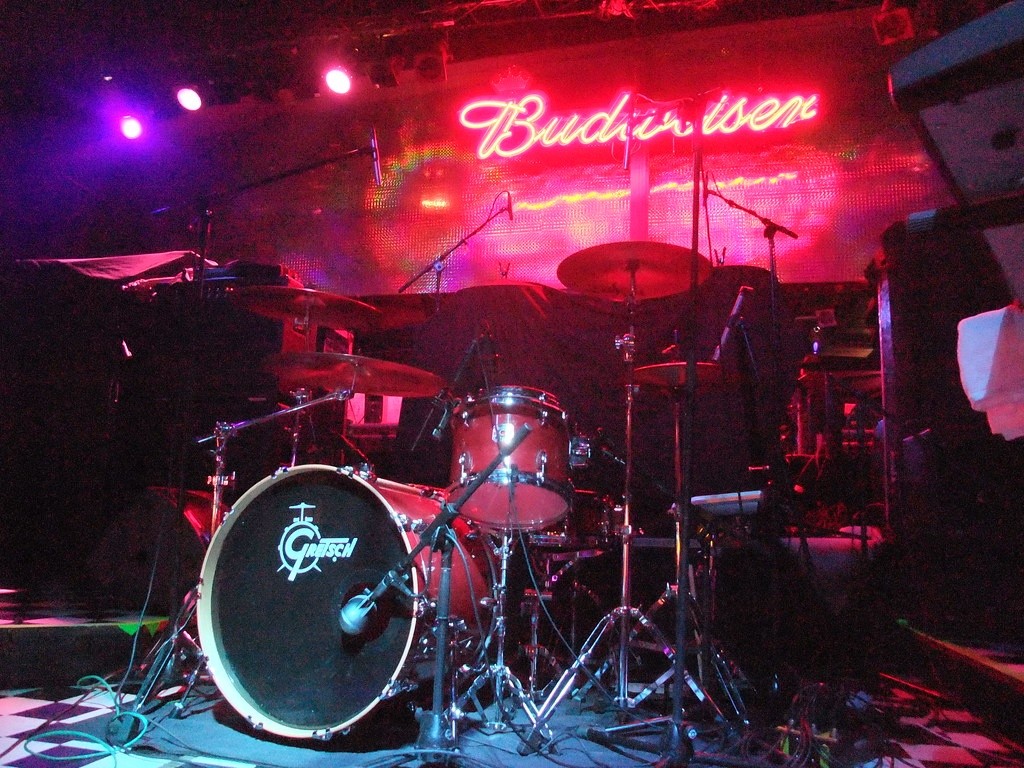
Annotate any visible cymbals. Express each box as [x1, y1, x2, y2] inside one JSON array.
[[556, 240, 715, 299], [258, 350, 447, 399], [223, 285, 384, 329], [633, 359, 721, 387]]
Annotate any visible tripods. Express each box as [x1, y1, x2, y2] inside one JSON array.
[[364, 261, 783, 767]]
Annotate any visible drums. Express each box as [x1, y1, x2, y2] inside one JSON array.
[[524, 487, 615, 560], [196, 462, 503, 740], [445, 383, 578, 531]]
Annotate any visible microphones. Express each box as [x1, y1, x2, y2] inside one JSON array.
[[703, 174, 709, 206], [337, 594, 378, 636], [711, 288, 744, 361], [623, 104, 635, 170], [507, 192, 513, 220], [453, 340, 478, 382], [369, 122, 382, 186]]
